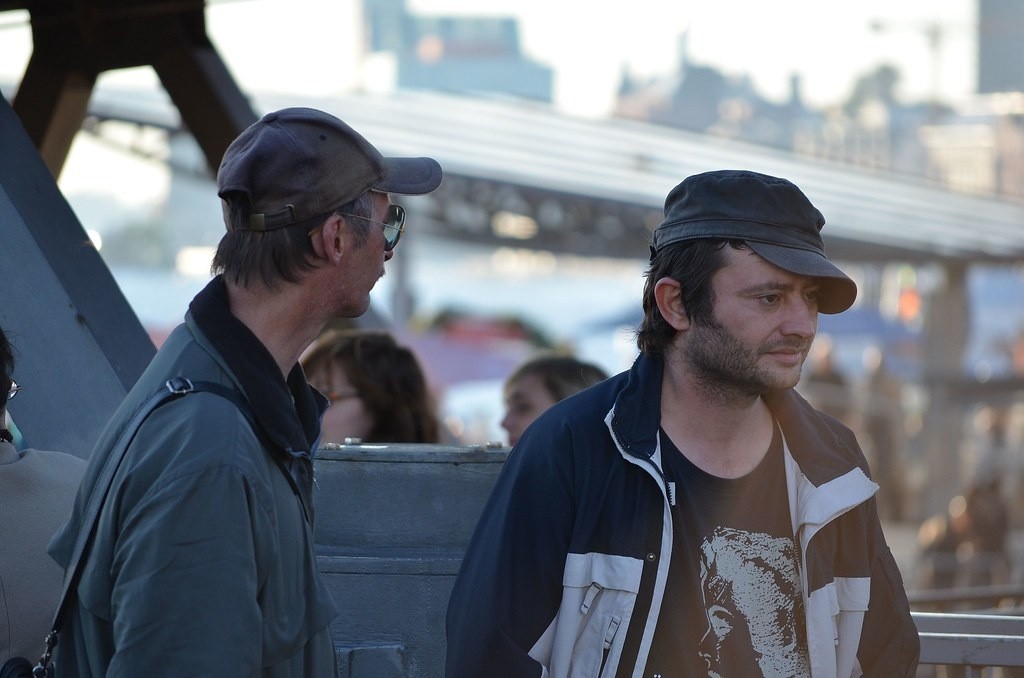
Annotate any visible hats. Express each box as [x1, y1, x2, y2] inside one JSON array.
[[217, 107, 442, 231], [650, 170, 857, 315]]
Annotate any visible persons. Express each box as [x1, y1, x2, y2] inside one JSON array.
[[446, 170, 920, 678], [0, 326, 88, 678], [292, 333, 438, 443], [495, 358, 604, 449], [37, 107, 445, 678], [782, 328, 1024, 678]]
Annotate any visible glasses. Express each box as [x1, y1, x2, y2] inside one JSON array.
[[7, 377, 22, 400], [308, 204, 405, 251]]
